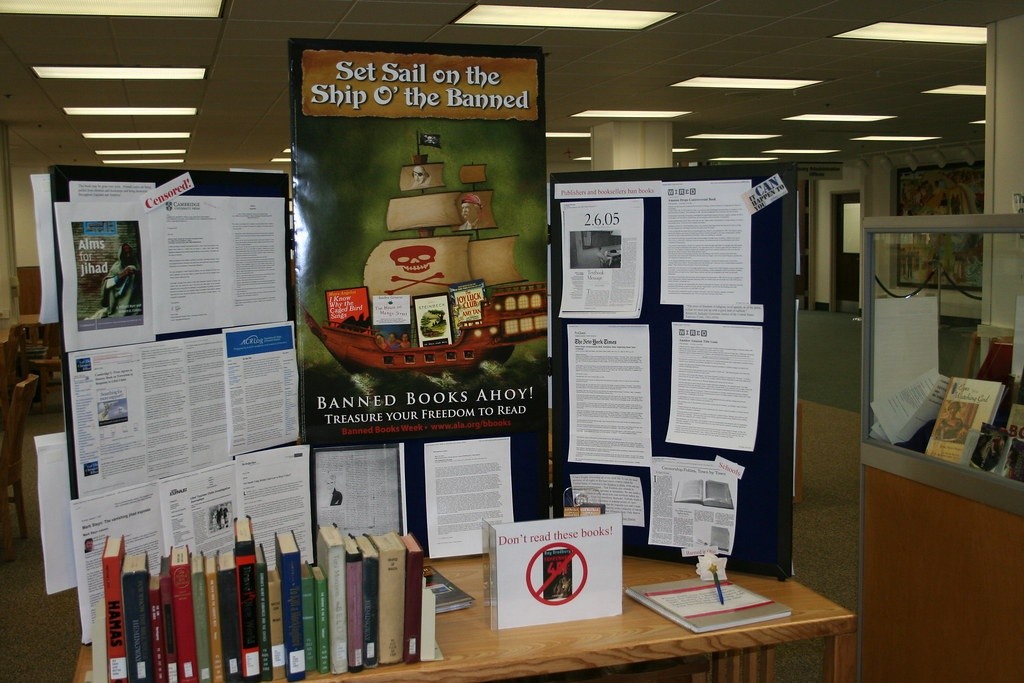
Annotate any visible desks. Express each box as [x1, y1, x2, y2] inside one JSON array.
[[74, 554, 857, 683]]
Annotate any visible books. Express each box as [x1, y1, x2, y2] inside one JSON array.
[[101, 515, 476, 683], [624, 577, 793, 634], [924, 376, 1005, 467]]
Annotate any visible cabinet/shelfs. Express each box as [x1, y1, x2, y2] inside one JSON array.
[[861, 214, 1024, 683]]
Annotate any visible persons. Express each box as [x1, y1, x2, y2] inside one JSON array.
[[99, 242, 143, 318], [85, 538, 93, 553]]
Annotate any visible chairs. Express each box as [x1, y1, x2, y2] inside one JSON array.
[[0, 323, 61, 562]]
